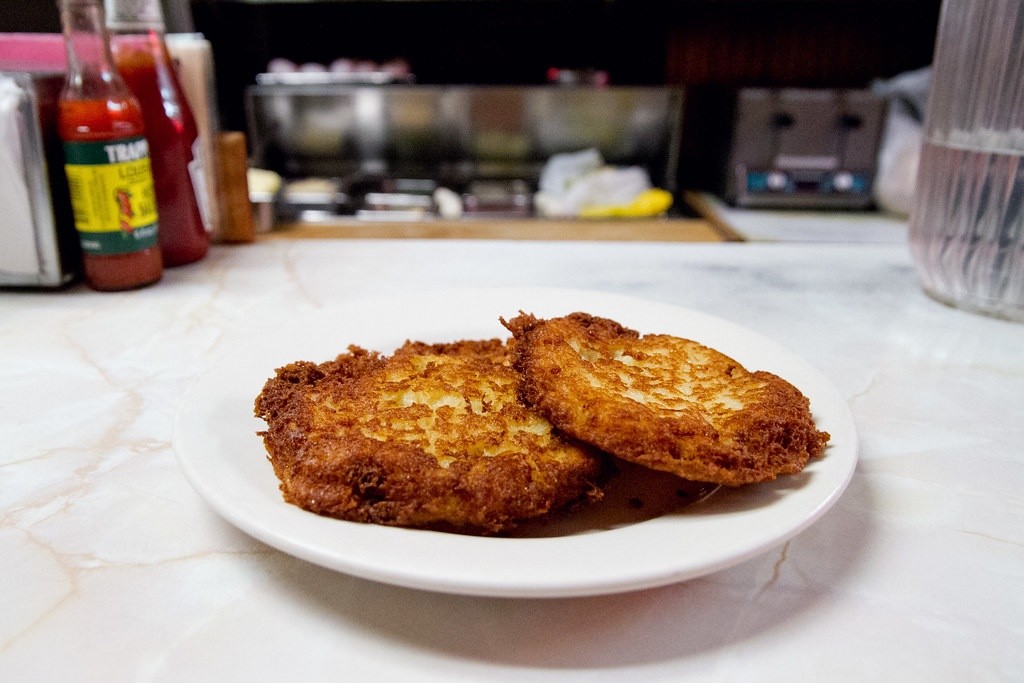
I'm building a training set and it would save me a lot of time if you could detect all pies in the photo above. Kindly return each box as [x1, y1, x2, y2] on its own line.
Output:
[247, 337, 615, 535]
[495, 309, 831, 485]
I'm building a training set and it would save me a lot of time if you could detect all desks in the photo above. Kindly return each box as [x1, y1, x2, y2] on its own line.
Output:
[0, 237, 1024, 683]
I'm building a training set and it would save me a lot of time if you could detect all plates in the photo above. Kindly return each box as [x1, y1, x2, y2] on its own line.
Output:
[171, 289, 862, 599]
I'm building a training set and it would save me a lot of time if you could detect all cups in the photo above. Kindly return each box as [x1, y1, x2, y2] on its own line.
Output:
[902, 0, 1023, 320]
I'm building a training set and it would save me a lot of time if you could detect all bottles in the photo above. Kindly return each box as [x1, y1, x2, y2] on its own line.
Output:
[54, 0, 164, 292]
[102, 0, 212, 267]
